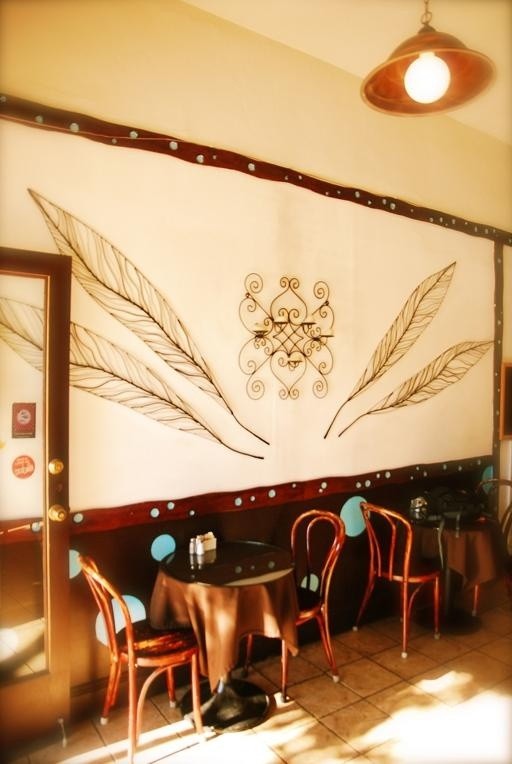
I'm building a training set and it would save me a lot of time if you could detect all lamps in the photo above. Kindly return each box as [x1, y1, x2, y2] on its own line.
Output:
[360, 0, 498, 118]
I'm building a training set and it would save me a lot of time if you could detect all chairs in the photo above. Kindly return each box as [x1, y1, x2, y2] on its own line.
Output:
[465, 479, 512, 617]
[76, 555, 205, 764]
[352, 502, 442, 659]
[244, 510, 345, 702]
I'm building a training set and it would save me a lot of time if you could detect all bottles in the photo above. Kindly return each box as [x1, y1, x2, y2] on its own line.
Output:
[197, 555, 205, 570]
[189, 554, 195, 570]
[189, 538, 195, 554]
[195, 540, 206, 555]
